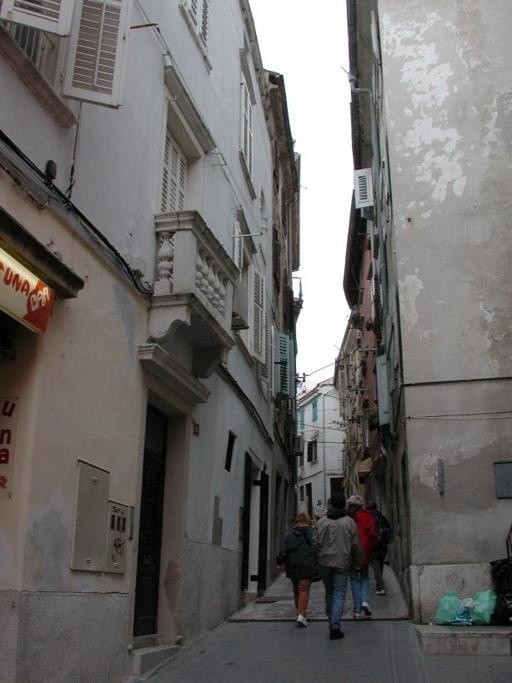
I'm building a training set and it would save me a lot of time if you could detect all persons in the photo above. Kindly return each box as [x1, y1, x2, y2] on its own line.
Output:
[308, 493, 362, 639]
[365, 500, 393, 595]
[345, 494, 381, 620]
[275, 510, 322, 626]
[312, 498, 328, 521]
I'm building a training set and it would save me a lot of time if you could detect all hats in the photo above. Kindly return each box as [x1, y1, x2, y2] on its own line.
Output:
[346, 495, 363, 505]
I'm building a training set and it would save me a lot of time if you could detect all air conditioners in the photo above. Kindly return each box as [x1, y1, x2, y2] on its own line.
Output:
[297, 436, 304, 456]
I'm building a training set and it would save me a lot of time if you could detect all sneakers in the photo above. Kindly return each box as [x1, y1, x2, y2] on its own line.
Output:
[376, 590, 384, 595]
[297, 614, 309, 626]
[354, 601, 373, 617]
[330, 630, 344, 639]
